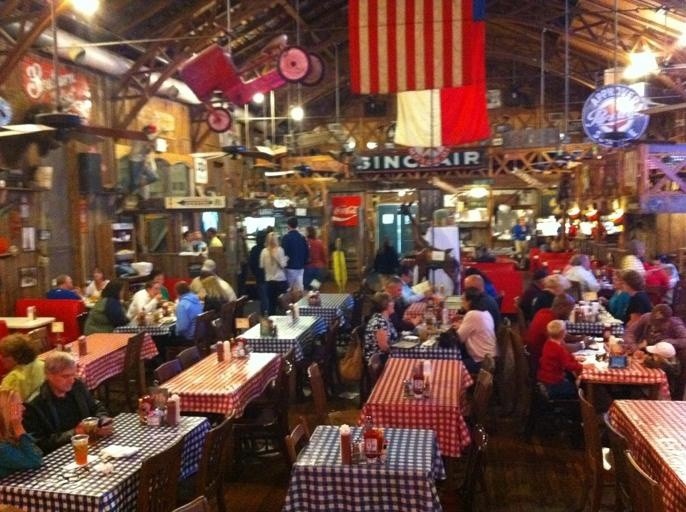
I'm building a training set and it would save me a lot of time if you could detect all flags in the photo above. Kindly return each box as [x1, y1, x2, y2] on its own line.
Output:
[390, 0, 489, 148]
[347, 0, 476, 98]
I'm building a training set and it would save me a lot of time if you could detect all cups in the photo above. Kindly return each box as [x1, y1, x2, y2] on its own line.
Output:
[72, 432, 89, 466]
[83, 415, 99, 444]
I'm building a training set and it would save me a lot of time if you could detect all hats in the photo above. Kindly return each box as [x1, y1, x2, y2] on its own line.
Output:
[201, 259, 218, 273]
[646, 341, 676, 358]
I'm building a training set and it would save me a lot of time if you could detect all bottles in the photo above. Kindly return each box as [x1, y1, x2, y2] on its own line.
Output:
[27, 304, 36, 320]
[413, 360, 424, 398]
[78, 335, 87, 355]
[339, 422, 352, 464]
[260, 309, 273, 337]
[574, 307, 586, 323]
[286, 302, 299, 323]
[424, 376, 432, 398]
[308, 290, 321, 305]
[603, 323, 612, 342]
[216, 340, 230, 362]
[56, 338, 65, 352]
[361, 414, 384, 458]
[402, 379, 410, 397]
[139, 392, 181, 427]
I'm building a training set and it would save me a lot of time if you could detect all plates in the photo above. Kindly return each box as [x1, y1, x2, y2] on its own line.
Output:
[403, 335, 418, 340]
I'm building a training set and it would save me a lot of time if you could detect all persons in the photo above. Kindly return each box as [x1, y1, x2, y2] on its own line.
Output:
[509, 218, 610, 267]
[355, 238, 502, 396]
[0, 384, 42, 480]
[232, 216, 328, 320]
[521, 237, 686, 409]
[25, 351, 107, 451]
[47, 227, 238, 369]
[0, 331, 46, 405]
